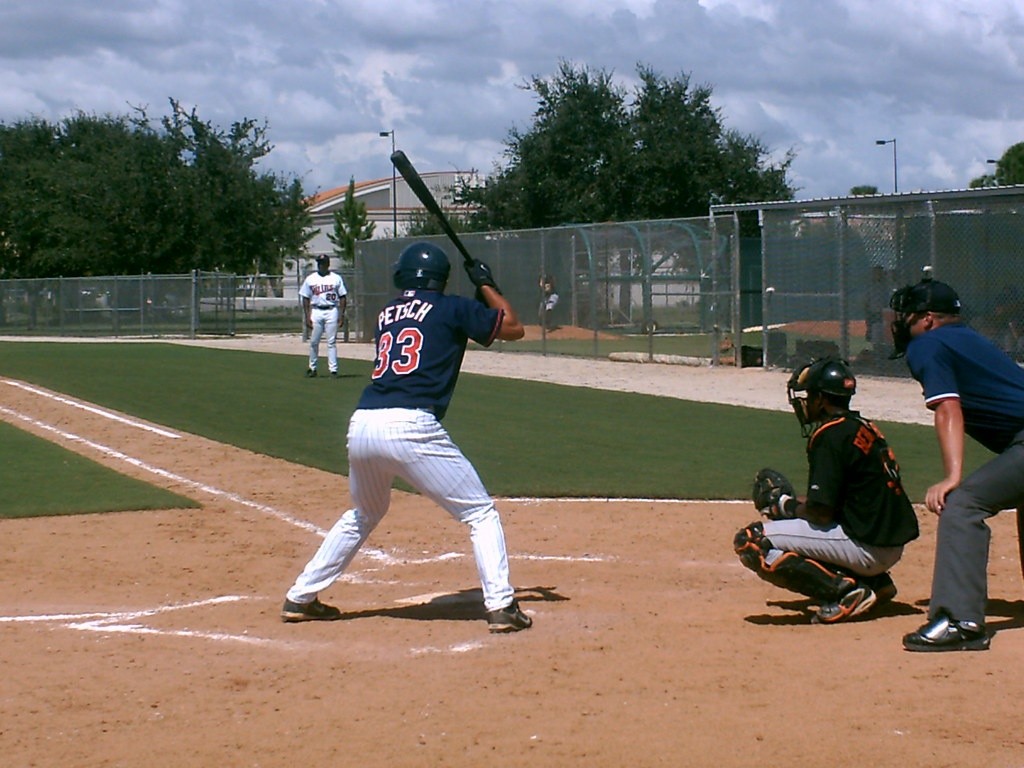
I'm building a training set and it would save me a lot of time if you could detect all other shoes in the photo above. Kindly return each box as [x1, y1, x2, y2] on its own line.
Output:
[304, 369, 317, 378]
[328, 371, 338, 381]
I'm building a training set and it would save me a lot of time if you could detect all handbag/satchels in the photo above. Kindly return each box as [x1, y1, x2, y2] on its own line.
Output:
[785, 339, 841, 369]
[735, 345, 762, 368]
[710, 335, 736, 366]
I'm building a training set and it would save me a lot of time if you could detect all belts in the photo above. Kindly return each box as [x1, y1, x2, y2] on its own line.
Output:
[317, 305, 335, 310]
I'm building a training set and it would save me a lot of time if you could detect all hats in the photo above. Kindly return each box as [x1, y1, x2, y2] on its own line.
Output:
[315, 254, 330, 263]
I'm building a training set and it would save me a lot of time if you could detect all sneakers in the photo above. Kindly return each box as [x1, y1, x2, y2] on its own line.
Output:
[856, 572, 897, 610]
[281, 597, 340, 622]
[903, 613, 990, 652]
[487, 598, 532, 632]
[819, 581, 878, 625]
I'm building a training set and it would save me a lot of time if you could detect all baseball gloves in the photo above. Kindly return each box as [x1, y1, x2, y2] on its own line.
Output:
[751, 467, 797, 519]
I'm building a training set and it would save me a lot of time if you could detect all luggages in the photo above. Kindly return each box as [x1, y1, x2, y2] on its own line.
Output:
[763, 327, 787, 369]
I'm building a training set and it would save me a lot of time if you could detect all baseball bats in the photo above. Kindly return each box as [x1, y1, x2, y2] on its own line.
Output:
[390, 149, 473, 265]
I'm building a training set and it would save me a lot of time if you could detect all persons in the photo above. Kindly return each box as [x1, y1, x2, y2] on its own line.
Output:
[863, 265, 884, 350]
[994, 284, 1024, 369]
[889, 279, 1024, 651]
[298, 254, 347, 379]
[280, 242, 532, 634]
[538, 273, 560, 332]
[733, 358, 920, 625]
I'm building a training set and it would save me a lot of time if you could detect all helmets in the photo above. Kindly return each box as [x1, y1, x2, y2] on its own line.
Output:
[392, 242, 451, 293]
[889, 277, 960, 314]
[787, 355, 856, 397]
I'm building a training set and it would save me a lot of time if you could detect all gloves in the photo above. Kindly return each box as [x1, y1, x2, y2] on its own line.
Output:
[463, 257, 503, 308]
